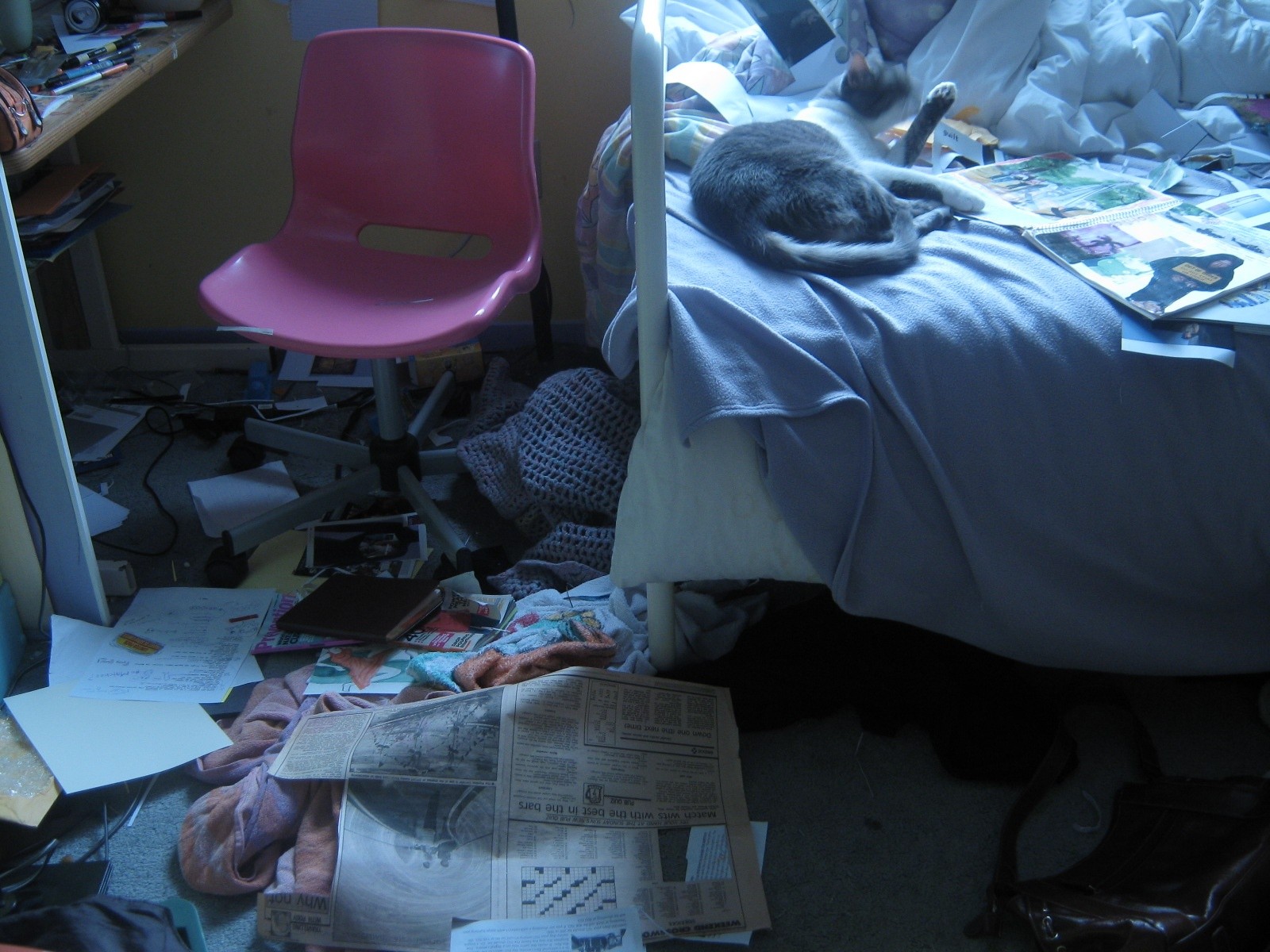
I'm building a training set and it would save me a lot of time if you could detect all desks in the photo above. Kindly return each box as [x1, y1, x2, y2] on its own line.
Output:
[0, 0, 271, 397]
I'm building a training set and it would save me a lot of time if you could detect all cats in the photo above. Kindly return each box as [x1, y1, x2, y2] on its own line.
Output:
[687, 51, 986, 277]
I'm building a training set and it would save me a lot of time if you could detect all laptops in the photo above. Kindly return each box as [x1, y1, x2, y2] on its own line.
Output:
[0, 860, 114, 899]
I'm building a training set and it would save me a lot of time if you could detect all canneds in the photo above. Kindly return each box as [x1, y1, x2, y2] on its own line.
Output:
[64, 0, 114, 34]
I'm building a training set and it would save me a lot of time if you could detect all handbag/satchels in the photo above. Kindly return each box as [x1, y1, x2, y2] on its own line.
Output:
[0, 67, 43, 155]
[981, 685, 1270, 952]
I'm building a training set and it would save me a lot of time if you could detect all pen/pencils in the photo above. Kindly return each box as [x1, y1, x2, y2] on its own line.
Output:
[117, 10, 203, 21]
[29, 33, 141, 97]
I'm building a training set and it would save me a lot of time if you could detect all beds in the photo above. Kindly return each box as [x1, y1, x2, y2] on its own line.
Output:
[600, 1, 1270, 680]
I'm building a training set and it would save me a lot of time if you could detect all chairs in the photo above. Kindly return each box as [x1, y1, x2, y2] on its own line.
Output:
[197, 28, 543, 586]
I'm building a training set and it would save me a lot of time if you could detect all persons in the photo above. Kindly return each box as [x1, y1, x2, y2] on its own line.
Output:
[1126, 253, 1244, 313]
[1181, 322, 1200, 339]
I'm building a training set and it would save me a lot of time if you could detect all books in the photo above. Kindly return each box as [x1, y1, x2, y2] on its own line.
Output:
[249, 589, 518, 655]
[937, 150, 1270, 338]
